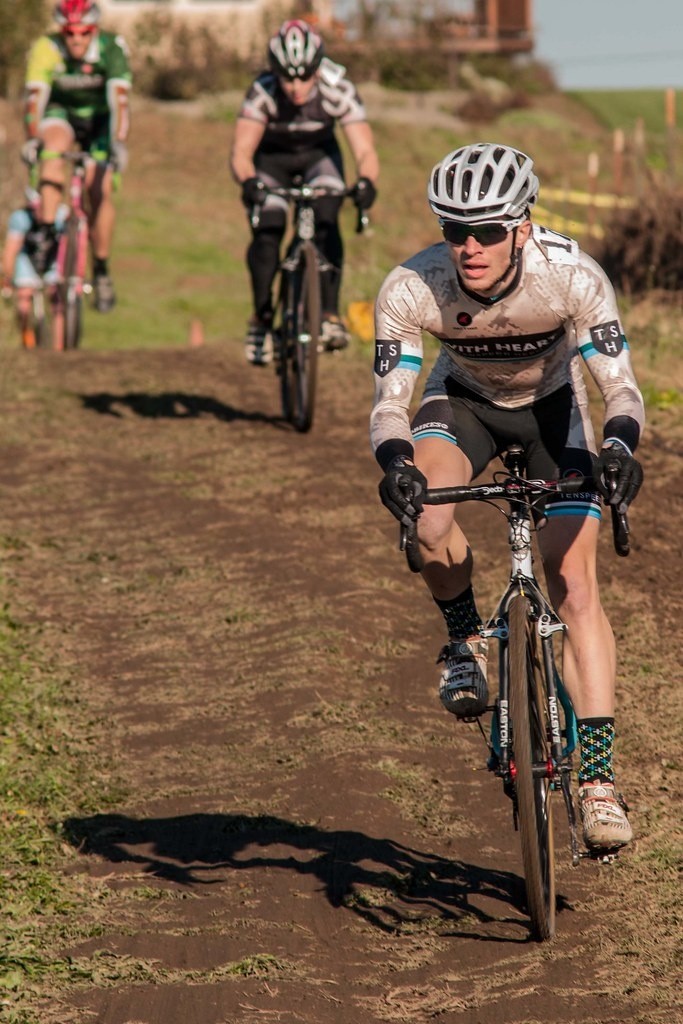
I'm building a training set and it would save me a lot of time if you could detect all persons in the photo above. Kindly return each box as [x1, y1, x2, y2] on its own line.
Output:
[230, 19, 378, 367]
[0, 190, 71, 334]
[368, 143, 644, 845]
[21, 0, 132, 311]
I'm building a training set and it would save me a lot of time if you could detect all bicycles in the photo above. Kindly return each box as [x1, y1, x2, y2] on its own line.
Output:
[391, 445, 635, 943]
[242, 180, 370, 434]
[3, 144, 121, 353]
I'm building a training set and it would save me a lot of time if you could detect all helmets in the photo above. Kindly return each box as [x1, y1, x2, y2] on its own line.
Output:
[267, 19, 324, 81]
[53, 0, 100, 25]
[428, 143, 539, 222]
[25, 185, 41, 208]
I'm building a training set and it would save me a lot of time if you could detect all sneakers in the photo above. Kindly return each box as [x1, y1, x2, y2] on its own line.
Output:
[245, 316, 274, 366]
[318, 318, 351, 349]
[435, 635, 488, 716]
[572, 779, 633, 849]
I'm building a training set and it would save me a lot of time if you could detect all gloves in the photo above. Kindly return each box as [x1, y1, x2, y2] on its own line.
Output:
[22, 137, 40, 166]
[594, 434, 644, 514]
[352, 176, 376, 209]
[109, 139, 127, 177]
[242, 178, 270, 209]
[374, 456, 427, 527]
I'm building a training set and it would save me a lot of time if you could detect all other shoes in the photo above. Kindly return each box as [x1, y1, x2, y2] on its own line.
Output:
[93, 274, 113, 312]
[30, 224, 55, 271]
[23, 323, 37, 348]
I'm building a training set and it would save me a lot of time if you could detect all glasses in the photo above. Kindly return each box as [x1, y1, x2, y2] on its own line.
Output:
[438, 211, 528, 246]
[60, 25, 94, 36]
